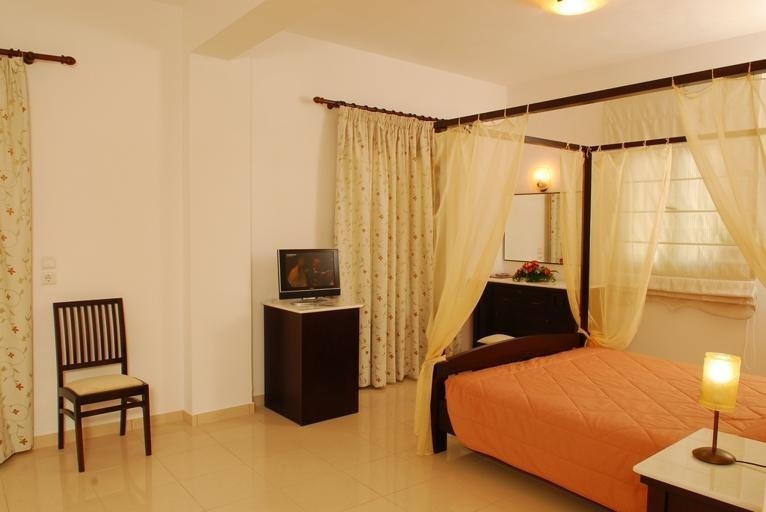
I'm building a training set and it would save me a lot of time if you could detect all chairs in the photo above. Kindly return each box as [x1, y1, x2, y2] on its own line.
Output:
[52, 297, 152, 471]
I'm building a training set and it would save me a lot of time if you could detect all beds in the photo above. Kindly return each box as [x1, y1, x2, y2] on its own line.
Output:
[430, 333, 766, 511]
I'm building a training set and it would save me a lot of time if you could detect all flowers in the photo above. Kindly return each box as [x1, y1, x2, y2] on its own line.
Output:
[511, 260, 559, 283]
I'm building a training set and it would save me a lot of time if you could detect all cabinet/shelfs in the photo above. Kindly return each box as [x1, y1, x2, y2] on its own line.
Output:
[262, 301, 365, 426]
[474, 275, 606, 349]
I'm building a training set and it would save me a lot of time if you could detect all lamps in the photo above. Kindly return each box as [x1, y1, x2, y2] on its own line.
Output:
[691, 351, 742, 465]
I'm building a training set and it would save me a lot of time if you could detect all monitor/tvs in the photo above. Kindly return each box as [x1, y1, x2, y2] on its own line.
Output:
[277, 249, 340, 305]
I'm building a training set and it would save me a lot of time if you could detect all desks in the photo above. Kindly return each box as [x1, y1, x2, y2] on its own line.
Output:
[632, 427, 766, 512]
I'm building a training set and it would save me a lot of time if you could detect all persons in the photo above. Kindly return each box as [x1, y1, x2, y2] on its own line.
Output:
[308, 258, 325, 287]
[286, 254, 307, 287]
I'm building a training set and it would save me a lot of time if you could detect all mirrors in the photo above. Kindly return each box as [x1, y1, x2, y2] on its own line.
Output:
[503, 192, 582, 264]
